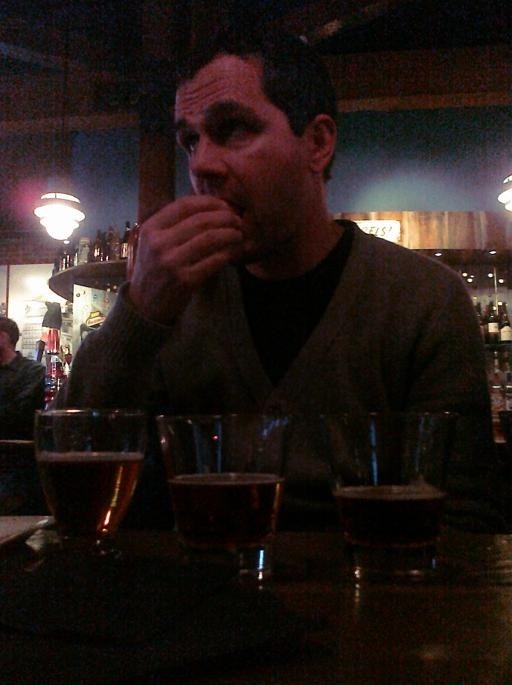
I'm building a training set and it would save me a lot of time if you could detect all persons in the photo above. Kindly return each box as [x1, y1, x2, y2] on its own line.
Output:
[46, 20, 505, 529]
[1, 316, 46, 439]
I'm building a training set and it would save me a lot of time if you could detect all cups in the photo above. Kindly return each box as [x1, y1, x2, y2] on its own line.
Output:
[29, 402, 143, 589]
[156, 407, 296, 591]
[317, 400, 456, 586]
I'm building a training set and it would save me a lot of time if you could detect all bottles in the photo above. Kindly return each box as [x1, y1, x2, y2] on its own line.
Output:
[476, 300, 511, 435]
[52, 220, 132, 273]
